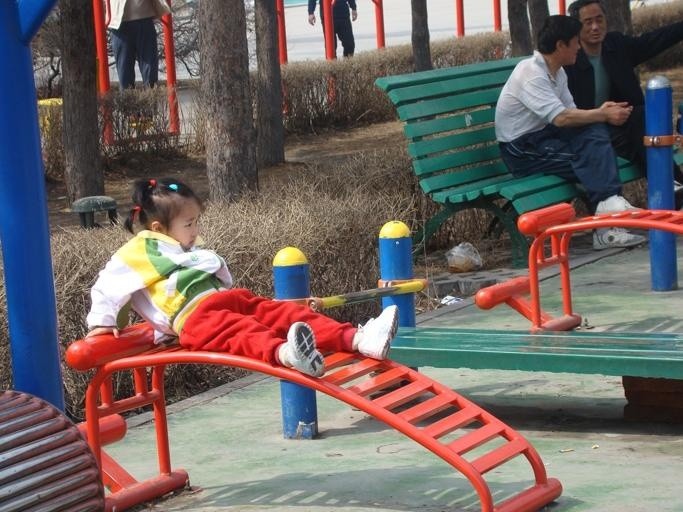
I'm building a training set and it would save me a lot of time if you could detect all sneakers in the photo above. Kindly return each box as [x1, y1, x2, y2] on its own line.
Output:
[592, 225, 646, 251]
[279, 321, 326, 378]
[594, 194, 644, 216]
[352, 304, 399, 361]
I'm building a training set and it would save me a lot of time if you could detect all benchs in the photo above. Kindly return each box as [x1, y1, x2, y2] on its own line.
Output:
[372, 55, 682, 268]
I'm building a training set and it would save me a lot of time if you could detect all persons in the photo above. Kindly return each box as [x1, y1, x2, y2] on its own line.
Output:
[495, 15, 645, 248]
[559, 1, 682, 211]
[106, 0, 171, 91]
[85, 177, 399, 377]
[308, 0, 357, 59]
